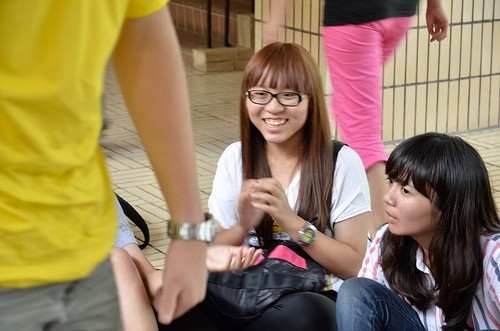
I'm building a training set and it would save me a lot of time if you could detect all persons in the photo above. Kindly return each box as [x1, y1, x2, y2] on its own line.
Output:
[157, 42, 373, 330]
[335, 132, 500, 331]
[109, 194, 165, 331]
[0, 0, 217, 331]
[322, 0, 448, 243]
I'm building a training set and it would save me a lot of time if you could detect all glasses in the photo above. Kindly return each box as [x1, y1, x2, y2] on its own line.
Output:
[244, 89, 305, 107]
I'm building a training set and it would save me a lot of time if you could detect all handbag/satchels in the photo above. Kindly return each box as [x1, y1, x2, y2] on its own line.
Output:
[206, 139, 348, 325]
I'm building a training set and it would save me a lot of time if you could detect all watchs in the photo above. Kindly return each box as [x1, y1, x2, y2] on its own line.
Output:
[166, 212, 216, 244]
[291, 220, 317, 247]
